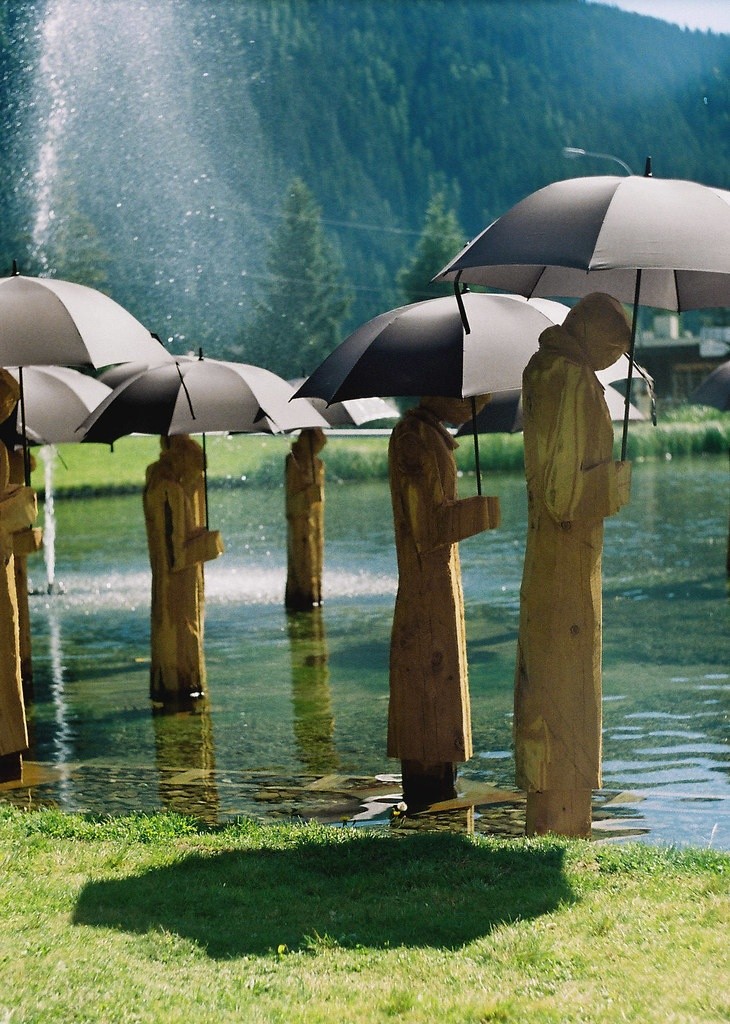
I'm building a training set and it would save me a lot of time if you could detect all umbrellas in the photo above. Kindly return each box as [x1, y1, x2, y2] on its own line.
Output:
[690, 361, 730, 412]
[73, 348, 335, 533]
[101, 355, 218, 388]
[226, 370, 403, 487]
[431, 154, 730, 462]
[288, 286, 607, 497]
[5, 367, 115, 444]
[0, 258, 147, 489]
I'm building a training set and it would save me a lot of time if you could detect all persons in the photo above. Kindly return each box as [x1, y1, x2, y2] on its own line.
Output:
[520, 291, 632, 838]
[0, 369, 47, 784]
[384, 389, 500, 821]
[142, 434, 221, 702]
[286, 428, 327, 607]
